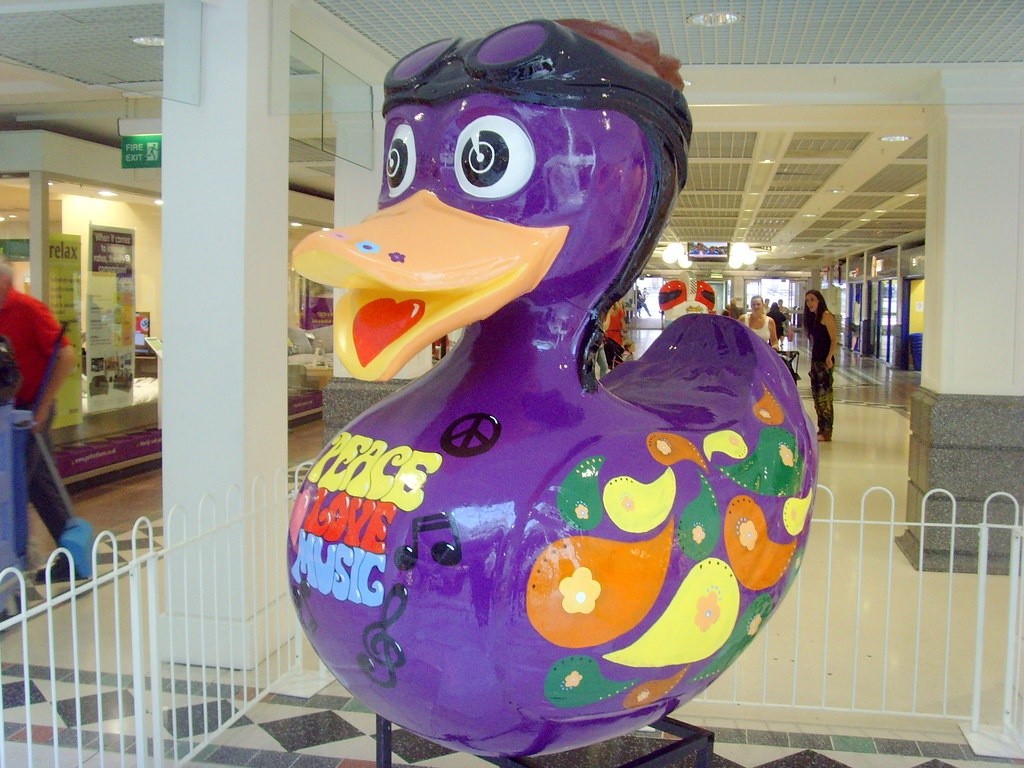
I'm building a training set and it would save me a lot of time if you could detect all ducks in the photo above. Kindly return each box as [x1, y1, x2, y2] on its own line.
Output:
[284, 19, 817, 756]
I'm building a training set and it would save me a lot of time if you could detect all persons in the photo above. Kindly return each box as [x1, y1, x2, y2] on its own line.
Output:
[1, 261, 77, 582]
[802, 289, 837, 442]
[596, 282, 653, 378]
[689, 242, 728, 256]
[723, 293, 797, 352]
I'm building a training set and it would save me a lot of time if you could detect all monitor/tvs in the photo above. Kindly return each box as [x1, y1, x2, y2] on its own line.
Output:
[688, 241, 730, 262]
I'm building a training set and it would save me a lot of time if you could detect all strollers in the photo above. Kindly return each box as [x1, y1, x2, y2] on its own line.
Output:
[602, 335, 625, 369]
[776, 351, 801, 388]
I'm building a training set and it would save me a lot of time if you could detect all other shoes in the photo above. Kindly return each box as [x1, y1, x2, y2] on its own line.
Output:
[818, 434, 831, 441]
[36, 559, 88, 583]
